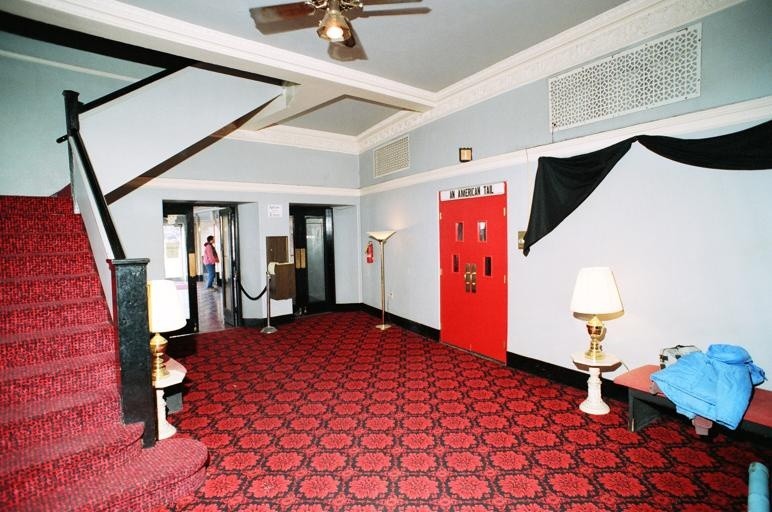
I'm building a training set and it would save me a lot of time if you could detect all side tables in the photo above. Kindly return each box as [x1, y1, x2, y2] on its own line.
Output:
[574, 354, 621, 416]
[151, 357, 187, 441]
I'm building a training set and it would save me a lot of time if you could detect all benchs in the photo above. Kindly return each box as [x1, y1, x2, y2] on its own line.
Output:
[611, 364, 772, 440]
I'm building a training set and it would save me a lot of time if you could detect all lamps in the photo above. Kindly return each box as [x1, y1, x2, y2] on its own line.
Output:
[316, 0, 351, 43]
[367, 231, 395, 331]
[146, 280, 187, 381]
[571, 266, 624, 358]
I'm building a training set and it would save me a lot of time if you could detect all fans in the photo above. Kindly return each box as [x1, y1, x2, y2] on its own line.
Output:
[249, 0, 431, 62]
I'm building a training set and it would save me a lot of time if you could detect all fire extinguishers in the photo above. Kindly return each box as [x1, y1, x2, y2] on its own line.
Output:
[365, 241, 373, 263]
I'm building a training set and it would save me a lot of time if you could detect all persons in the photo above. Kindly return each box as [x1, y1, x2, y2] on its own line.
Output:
[203, 235, 220, 292]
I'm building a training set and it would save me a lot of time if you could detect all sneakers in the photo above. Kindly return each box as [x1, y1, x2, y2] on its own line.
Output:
[207, 288, 218, 292]
